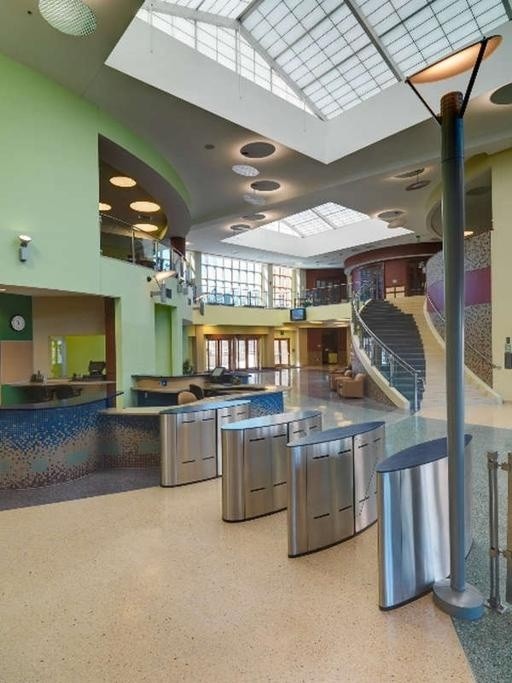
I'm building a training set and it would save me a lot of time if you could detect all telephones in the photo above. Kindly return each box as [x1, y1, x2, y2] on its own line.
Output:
[32, 370, 44, 382]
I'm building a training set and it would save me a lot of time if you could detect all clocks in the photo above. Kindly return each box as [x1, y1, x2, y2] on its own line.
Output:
[10, 313, 26, 332]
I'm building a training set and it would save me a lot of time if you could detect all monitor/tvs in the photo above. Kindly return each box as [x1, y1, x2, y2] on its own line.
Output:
[290, 308, 306, 321]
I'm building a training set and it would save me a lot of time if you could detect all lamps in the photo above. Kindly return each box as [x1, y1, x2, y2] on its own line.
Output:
[193, 294, 207, 316]
[151, 270, 176, 304]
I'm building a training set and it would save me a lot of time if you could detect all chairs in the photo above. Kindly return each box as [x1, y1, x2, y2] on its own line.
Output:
[55, 383, 73, 400]
[328, 365, 366, 400]
[177, 391, 197, 406]
[189, 383, 204, 400]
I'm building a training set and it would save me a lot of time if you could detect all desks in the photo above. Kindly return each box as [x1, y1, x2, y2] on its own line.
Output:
[9, 374, 114, 401]
